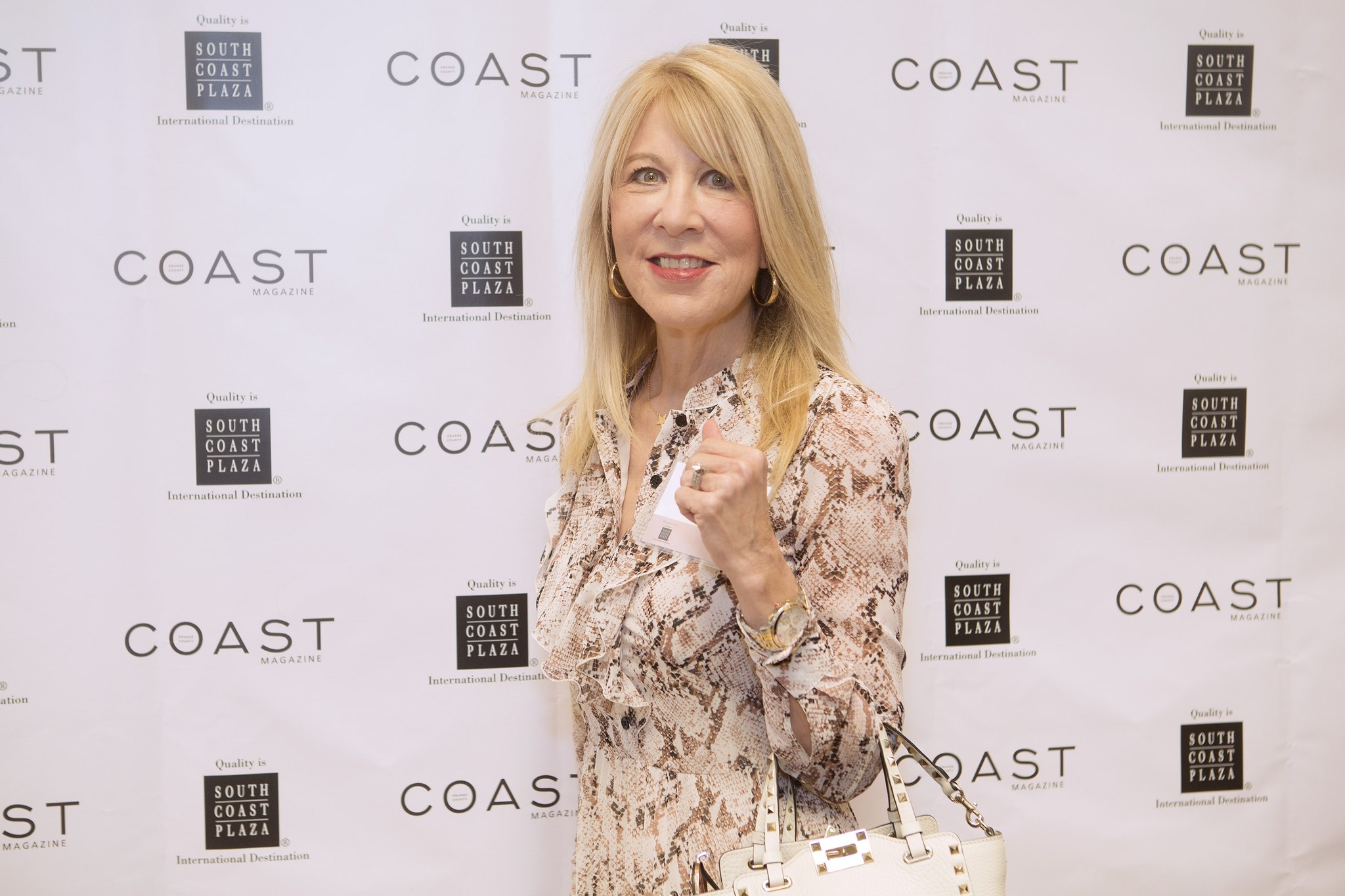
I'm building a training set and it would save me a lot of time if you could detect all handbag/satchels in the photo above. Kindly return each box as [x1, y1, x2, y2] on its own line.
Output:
[691, 714, 1008, 896]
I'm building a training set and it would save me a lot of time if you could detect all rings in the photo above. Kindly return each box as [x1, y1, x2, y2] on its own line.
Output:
[692, 463, 715, 490]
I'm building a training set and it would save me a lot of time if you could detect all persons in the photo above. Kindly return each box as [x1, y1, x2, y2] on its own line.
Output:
[532, 43, 910, 896]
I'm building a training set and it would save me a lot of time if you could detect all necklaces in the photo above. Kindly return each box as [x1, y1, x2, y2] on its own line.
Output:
[648, 351, 668, 429]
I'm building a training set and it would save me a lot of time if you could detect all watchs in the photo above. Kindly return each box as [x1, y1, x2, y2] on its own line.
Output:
[740, 582, 809, 652]
